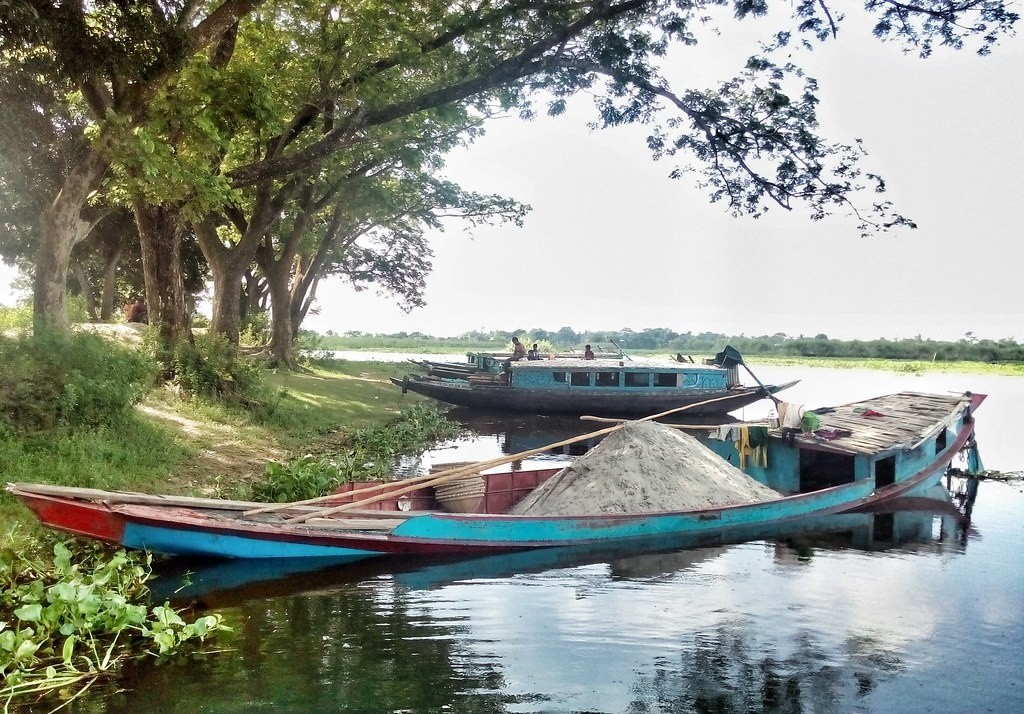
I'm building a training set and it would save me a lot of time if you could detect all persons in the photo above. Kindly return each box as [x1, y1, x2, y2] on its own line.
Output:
[527, 344, 540, 360]
[500, 337, 525, 375]
[129, 297, 147, 322]
[585, 345, 594, 360]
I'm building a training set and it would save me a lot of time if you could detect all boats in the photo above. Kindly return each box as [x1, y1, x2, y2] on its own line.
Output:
[389, 351, 803, 414]
[5, 389, 990, 565]
